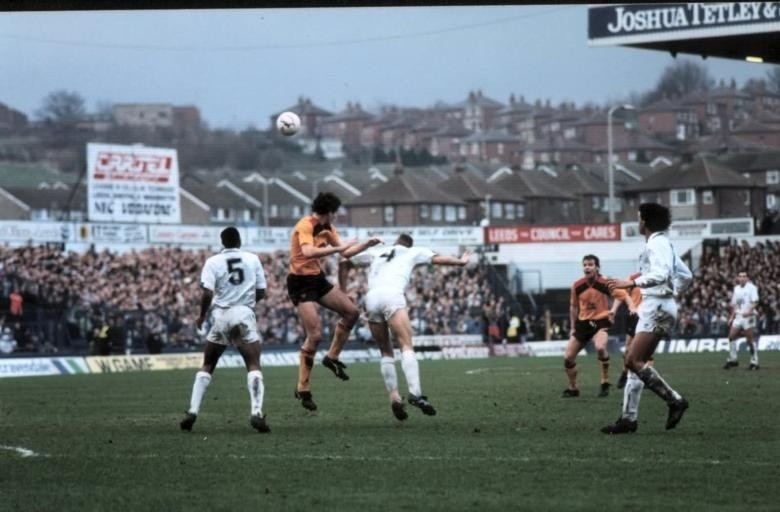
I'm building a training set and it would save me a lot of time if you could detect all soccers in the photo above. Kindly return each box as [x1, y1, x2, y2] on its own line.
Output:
[276, 111, 300, 135]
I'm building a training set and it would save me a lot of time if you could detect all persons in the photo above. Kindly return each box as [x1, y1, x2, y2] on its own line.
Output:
[564, 256, 642, 399]
[668, 238, 780, 370]
[287, 192, 385, 410]
[255, 251, 371, 343]
[339, 233, 474, 420]
[1, 237, 210, 359]
[600, 203, 689, 434]
[407, 250, 571, 357]
[180, 227, 270, 432]
[607, 273, 655, 388]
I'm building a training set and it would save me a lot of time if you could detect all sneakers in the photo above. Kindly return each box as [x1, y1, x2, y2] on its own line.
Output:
[408, 393, 436, 415]
[722, 360, 740, 370]
[178, 412, 196, 432]
[248, 413, 271, 435]
[598, 381, 610, 397]
[748, 363, 759, 370]
[601, 418, 639, 435]
[293, 386, 318, 411]
[667, 397, 688, 432]
[323, 356, 350, 381]
[561, 388, 580, 397]
[392, 399, 408, 420]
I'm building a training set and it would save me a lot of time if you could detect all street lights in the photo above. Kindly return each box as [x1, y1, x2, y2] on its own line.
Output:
[607, 100, 639, 222]
